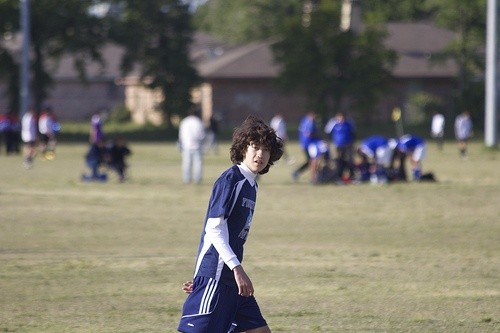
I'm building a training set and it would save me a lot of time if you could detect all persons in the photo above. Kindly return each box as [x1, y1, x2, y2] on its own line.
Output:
[175, 114, 284, 332]
[19, 106, 38, 168]
[431, 109, 446, 151]
[178, 106, 205, 186]
[291, 110, 427, 186]
[38, 105, 58, 157]
[455, 109, 473, 157]
[271, 112, 297, 166]
